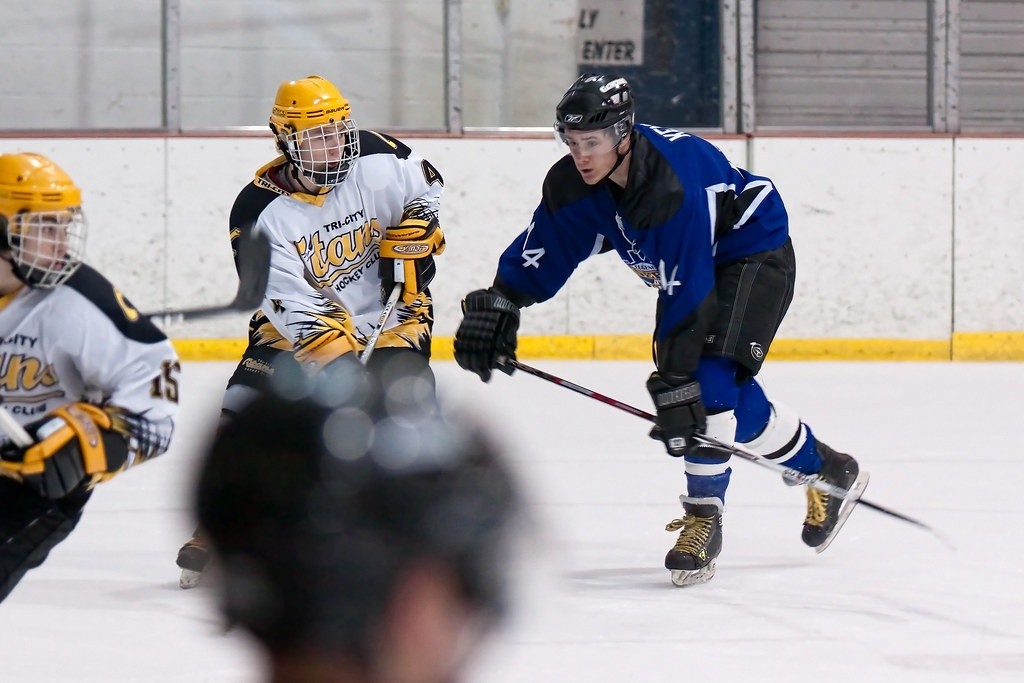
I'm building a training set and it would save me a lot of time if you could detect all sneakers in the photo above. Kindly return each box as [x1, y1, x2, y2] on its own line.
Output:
[176, 523, 214, 589]
[664, 494, 724, 586]
[801, 434, 870, 554]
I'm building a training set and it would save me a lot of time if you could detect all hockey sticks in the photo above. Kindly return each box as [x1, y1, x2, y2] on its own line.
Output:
[498, 355, 932, 536]
[0, 406, 34, 448]
[359, 280, 406, 363]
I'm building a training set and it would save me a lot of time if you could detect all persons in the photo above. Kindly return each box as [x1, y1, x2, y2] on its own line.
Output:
[177, 76, 446, 590]
[193, 369, 519, 683]
[0, 150, 180, 600]
[454, 71, 869, 588]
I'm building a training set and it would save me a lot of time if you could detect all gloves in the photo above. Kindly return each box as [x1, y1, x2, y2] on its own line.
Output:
[378, 224, 436, 308]
[647, 371, 707, 457]
[453, 289, 520, 383]
[0, 402, 128, 499]
[300, 334, 365, 380]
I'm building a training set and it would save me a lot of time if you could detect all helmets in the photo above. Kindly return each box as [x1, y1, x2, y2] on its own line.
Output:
[197, 380, 512, 612]
[270, 76, 351, 183]
[0, 152, 82, 289]
[555, 72, 634, 131]
